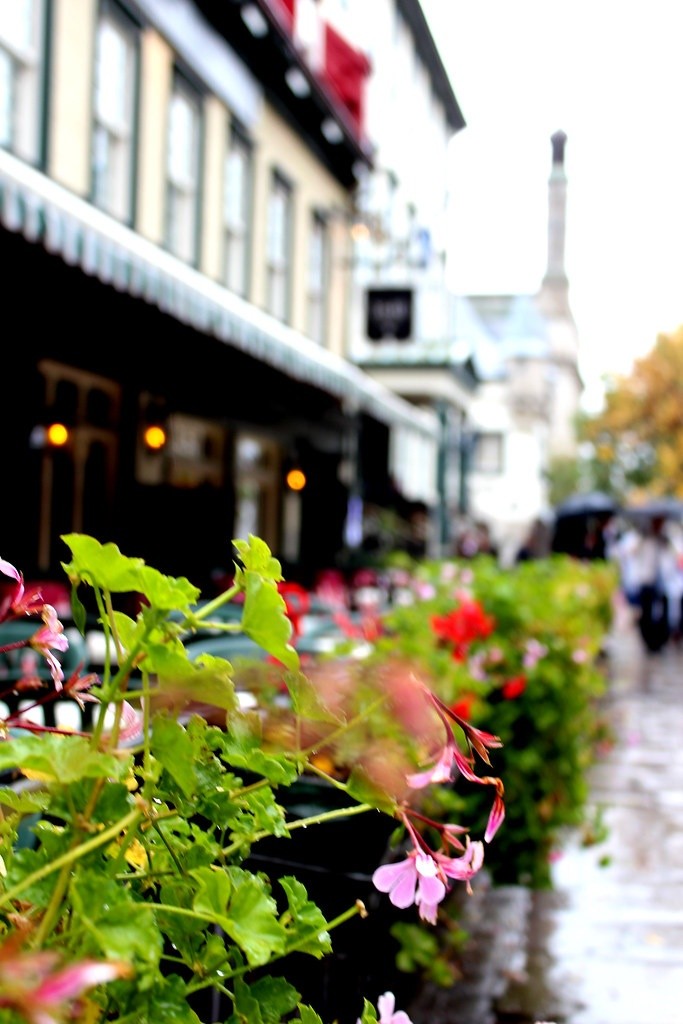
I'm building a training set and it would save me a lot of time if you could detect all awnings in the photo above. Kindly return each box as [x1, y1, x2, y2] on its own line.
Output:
[0, 147, 442, 445]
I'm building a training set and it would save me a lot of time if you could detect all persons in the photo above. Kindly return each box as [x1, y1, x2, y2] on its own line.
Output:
[428, 501, 682, 644]
